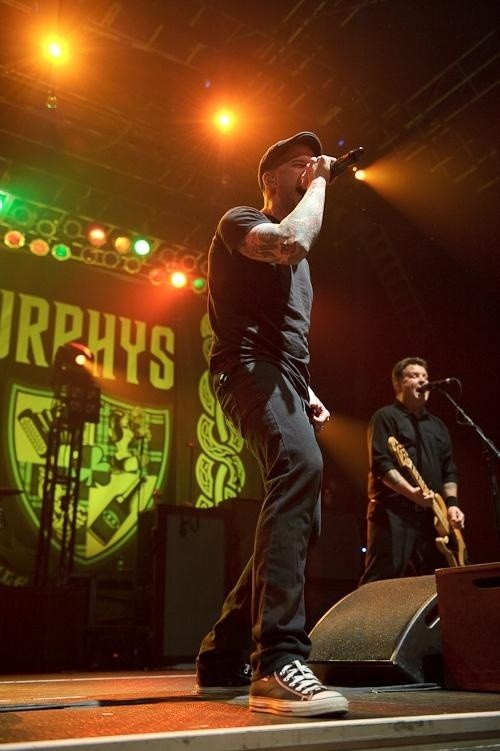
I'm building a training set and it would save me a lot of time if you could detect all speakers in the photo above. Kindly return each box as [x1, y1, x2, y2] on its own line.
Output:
[435, 562, 500, 693]
[303, 574, 440, 687]
[136, 504, 234, 665]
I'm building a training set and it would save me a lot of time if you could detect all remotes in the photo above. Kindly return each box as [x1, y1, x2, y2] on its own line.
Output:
[423, 377, 454, 391]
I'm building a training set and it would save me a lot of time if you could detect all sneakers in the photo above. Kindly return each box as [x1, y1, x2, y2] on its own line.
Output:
[249, 659, 349, 717]
[196, 662, 251, 694]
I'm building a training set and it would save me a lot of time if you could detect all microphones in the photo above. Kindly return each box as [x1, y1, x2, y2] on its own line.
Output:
[329, 147, 363, 183]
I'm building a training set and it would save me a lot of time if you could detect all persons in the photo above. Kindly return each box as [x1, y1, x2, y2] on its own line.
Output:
[194, 128, 352, 719]
[356, 357, 469, 587]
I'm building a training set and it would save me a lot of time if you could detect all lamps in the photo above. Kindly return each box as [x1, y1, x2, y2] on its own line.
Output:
[0, 157, 210, 302]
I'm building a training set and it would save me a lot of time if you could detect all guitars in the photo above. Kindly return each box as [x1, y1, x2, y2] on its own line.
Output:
[387, 436, 466, 567]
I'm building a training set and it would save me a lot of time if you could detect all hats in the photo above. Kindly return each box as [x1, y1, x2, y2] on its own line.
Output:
[257, 131, 322, 190]
